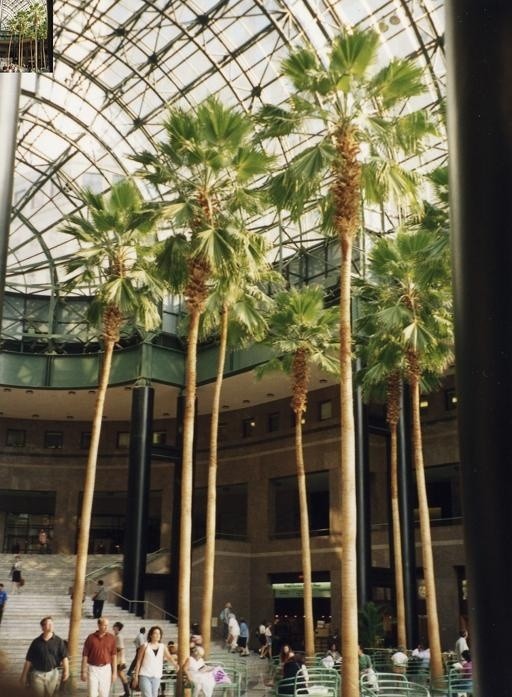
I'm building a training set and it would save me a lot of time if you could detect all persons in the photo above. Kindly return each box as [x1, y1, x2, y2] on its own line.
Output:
[112, 621, 131, 696]
[167, 637, 215, 697]
[127, 625, 147, 675]
[257, 619, 272, 660]
[20, 615, 70, 696]
[8, 554, 25, 594]
[455, 630, 472, 681]
[91, 580, 108, 615]
[1, 582, 8, 623]
[67, 578, 88, 606]
[266, 644, 309, 694]
[358, 644, 379, 692]
[80, 616, 118, 696]
[11, 525, 127, 553]
[391, 642, 430, 685]
[325, 643, 342, 667]
[131, 625, 179, 696]
[224, 602, 250, 657]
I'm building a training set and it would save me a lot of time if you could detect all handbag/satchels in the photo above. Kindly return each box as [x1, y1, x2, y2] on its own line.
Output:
[130, 675, 140, 690]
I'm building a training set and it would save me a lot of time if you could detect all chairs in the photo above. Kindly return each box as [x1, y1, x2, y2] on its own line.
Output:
[149, 641, 477, 696]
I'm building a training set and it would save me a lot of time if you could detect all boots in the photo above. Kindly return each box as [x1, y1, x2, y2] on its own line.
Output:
[119, 681, 130, 696]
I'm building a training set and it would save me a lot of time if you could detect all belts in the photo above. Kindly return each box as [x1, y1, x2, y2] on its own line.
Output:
[92, 662, 105, 666]
